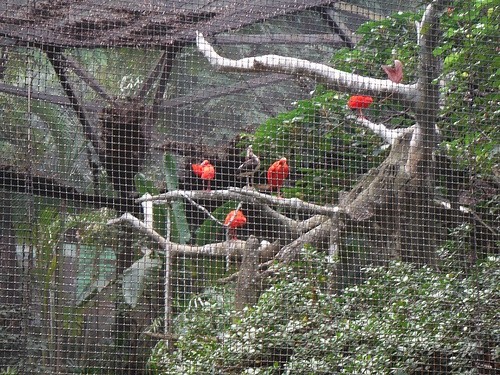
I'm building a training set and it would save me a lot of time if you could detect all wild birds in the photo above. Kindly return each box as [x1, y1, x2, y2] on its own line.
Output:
[192, 159, 216, 191]
[267, 157, 289, 196]
[381, 60, 404, 84]
[224, 210, 247, 240]
[348, 95, 374, 119]
[236, 144, 261, 189]
